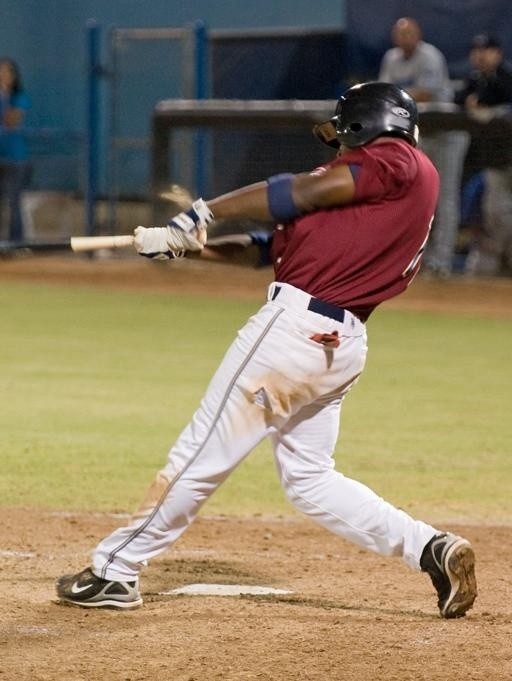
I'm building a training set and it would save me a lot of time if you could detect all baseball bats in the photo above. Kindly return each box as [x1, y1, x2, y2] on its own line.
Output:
[0, 235, 136, 263]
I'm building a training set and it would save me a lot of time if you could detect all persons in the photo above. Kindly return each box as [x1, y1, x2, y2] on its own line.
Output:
[455, 32, 511, 279]
[51, 80, 478, 623]
[376, 14, 472, 279]
[1, 55, 37, 264]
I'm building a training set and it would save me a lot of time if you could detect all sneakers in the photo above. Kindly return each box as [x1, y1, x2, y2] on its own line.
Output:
[419, 531, 480, 620]
[55, 566, 144, 609]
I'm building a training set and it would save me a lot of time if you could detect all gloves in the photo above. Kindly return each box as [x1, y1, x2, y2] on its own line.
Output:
[166, 197, 215, 252]
[133, 225, 188, 262]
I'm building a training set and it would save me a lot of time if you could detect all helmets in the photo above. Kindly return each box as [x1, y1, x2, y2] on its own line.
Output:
[312, 81, 421, 149]
